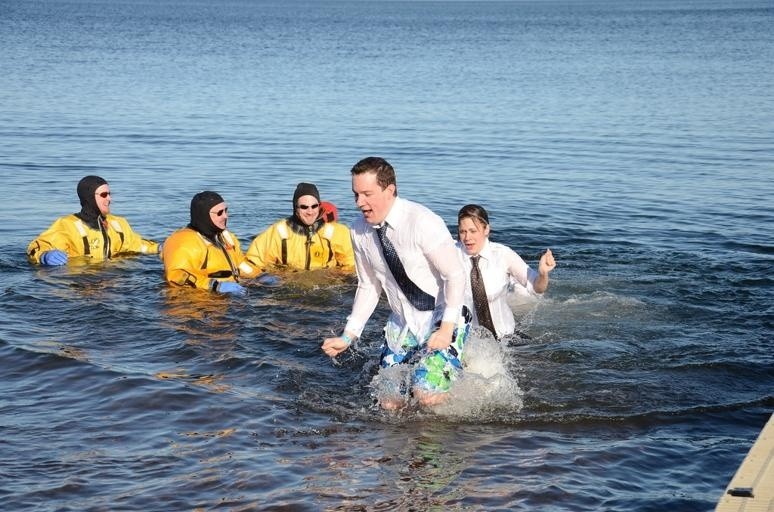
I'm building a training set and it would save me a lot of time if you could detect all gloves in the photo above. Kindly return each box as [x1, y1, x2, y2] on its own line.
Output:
[210, 280, 247, 297]
[40, 251, 68, 266]
[256, 272, 281, 285]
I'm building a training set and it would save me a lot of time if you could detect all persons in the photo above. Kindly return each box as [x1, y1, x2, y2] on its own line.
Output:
[160, 191, 280, 297]
[455, 204, 556, 341]
[247, 183, 354, 275]
[527, 254, 531, 254]
[321, 157, 473, 413]
[26, 176, 163, 266]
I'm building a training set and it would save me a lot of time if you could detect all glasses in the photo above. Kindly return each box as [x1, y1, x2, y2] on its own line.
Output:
[298, 204, 318, 209]
[210, 207, 227, 216]
[95, 192, 110, 198]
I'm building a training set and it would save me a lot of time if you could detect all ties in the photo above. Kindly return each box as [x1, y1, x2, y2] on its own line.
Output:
[376, 224, 436, 312]
[470, 255, 497, 340]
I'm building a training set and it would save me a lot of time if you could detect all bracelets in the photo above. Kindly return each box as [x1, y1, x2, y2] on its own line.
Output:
[340, 334, 352, 345]
